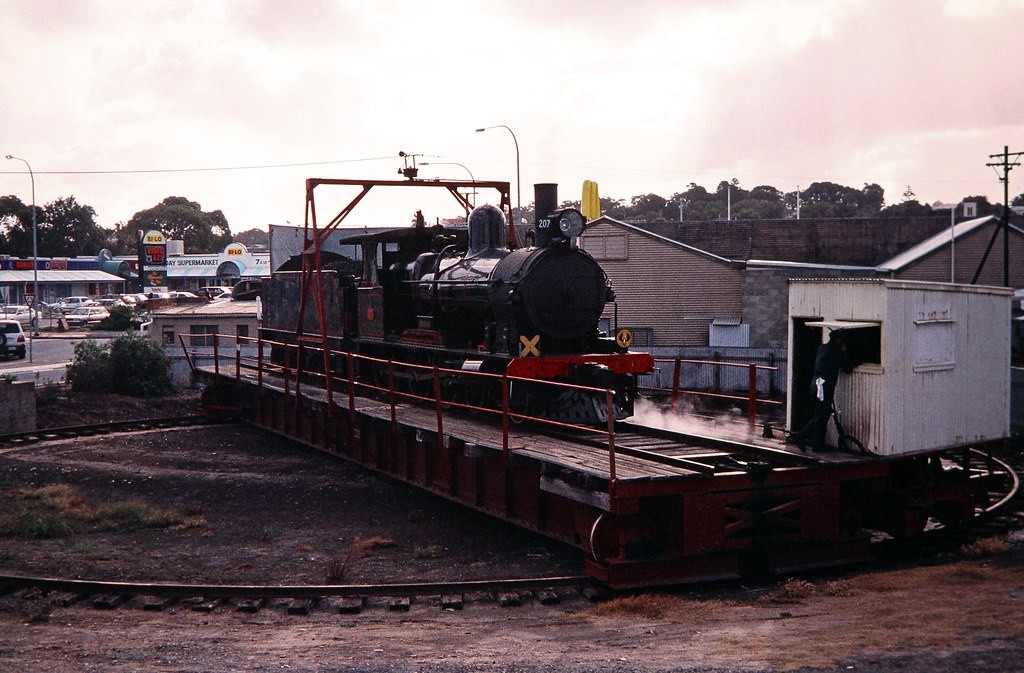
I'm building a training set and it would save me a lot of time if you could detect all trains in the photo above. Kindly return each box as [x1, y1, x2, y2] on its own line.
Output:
[266, 183, 656, 433]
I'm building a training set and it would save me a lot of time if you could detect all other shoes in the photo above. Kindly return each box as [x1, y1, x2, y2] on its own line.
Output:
[812, 445, 826, 452]
[792, 432, 806, 452]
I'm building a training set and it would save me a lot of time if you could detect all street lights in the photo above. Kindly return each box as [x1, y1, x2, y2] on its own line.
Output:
[419, 161, 476, 211]
[6, 155, 39, 337]
[477, 125, 523, 224]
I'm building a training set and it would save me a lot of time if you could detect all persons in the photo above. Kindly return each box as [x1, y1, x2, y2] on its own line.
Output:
[792, 330, 856, 452]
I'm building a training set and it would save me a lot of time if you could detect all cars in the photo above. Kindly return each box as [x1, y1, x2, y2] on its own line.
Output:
[0, 286, 234, 360]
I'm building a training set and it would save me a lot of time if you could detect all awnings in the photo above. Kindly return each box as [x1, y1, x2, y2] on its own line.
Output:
[0, 270, 127, 305]
